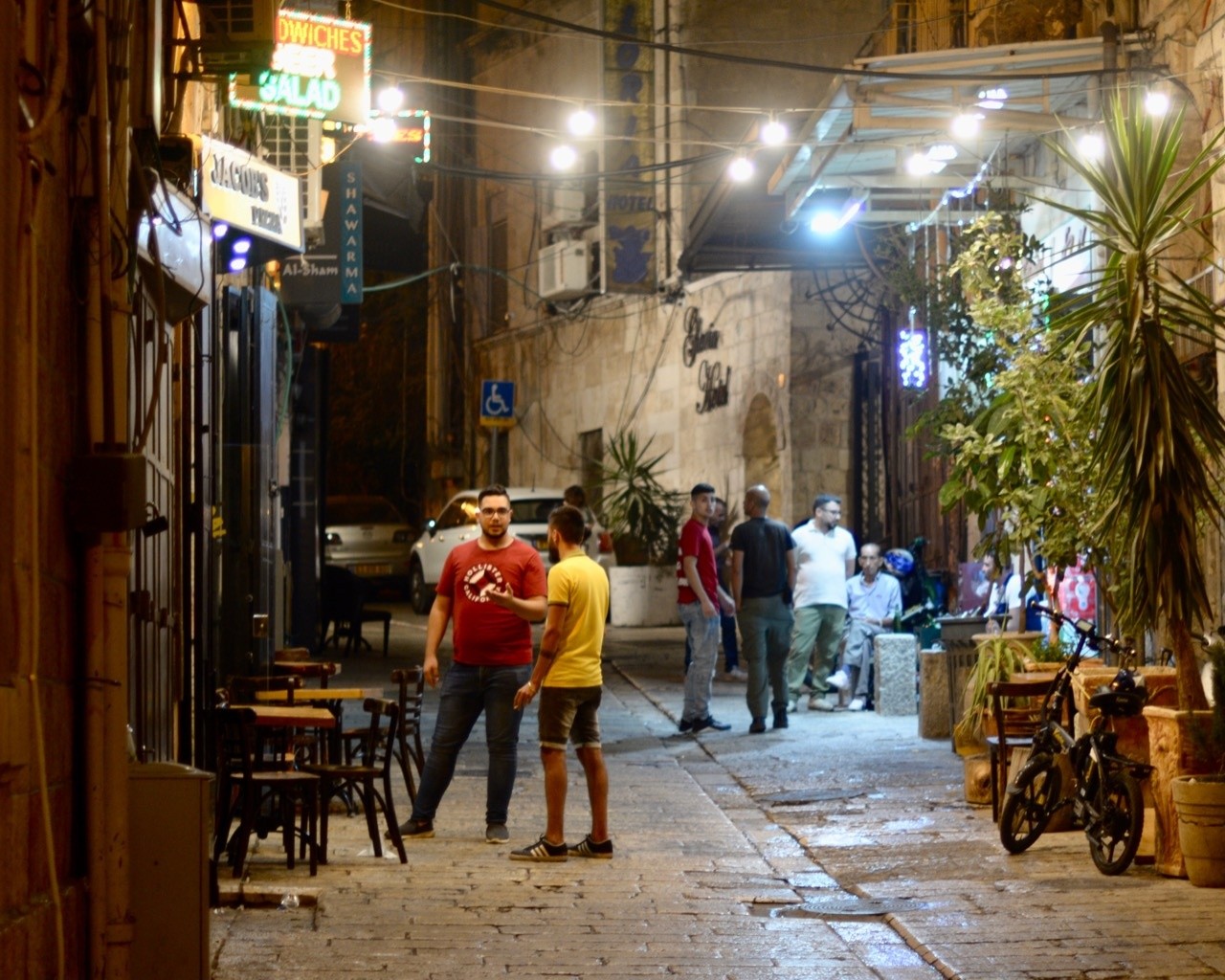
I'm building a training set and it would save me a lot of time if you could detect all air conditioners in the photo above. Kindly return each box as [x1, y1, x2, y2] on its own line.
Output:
[257, 111, 323, 228]
[539, 168, 585, 234]
[538, 238, 590, 301]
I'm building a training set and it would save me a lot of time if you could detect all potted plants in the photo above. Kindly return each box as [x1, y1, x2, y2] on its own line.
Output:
[591, 432, 689, 628]
[936, 69, 1225, 879]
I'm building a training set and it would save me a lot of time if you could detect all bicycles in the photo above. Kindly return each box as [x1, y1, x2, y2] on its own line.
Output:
[997, 598, 1156, 876]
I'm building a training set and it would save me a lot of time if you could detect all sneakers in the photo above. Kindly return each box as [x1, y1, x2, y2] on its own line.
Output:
[568, 835, 616, 860]
[507, 833, 569, 862]
[384, 818, 435, 841]
[484, 823, 511, 846]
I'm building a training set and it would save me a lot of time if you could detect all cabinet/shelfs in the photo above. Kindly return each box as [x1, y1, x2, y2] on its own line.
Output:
[129, 759, 216, 980]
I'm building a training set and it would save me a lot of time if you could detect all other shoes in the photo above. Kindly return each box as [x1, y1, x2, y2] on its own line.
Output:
[786, 699, 799, 713]
[679, 715, 696, 733]
[747, 720, 768, 735]
[773, 716, 789, 729]
[806, 697, 834, 713]
[695, 715, 734, 736]
[825, 669, 853, 692]
[724, 668, 748, 682]
[847, 697, 866, 711]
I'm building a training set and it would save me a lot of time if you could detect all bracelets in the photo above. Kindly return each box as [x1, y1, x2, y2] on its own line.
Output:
[528, 681, 538, 694]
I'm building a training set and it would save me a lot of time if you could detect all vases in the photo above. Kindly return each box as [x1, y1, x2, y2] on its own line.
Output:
[1170, 773, 1225, 888]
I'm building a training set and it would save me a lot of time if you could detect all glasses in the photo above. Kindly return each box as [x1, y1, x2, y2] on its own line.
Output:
[482, 506, 510, 519]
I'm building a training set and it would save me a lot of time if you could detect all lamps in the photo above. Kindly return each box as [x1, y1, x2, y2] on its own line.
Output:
[907, 82, 1008, 173]
[808, 190, 872, 242]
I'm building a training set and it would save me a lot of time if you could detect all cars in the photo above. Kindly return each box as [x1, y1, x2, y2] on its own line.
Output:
[407, 485, 617, 623]
[322, 492, 417, 594]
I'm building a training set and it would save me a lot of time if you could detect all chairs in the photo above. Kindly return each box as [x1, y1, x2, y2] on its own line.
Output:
[316, 564, 393, 661]
[212, 648, 428, 881]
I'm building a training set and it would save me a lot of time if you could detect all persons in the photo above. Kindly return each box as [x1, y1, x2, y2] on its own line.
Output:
[983, 551, 1042, 637]
[675, 483, 901, 734]
[383, 484, 547, 843]
[560, 486, 605, 564]
[509, 505, 613, 860]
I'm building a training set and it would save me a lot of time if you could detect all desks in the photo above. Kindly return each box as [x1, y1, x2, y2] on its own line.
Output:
[275, 661, 342, 763]
[229, 707, 336, 862]
[254, 685, 383, 781]
